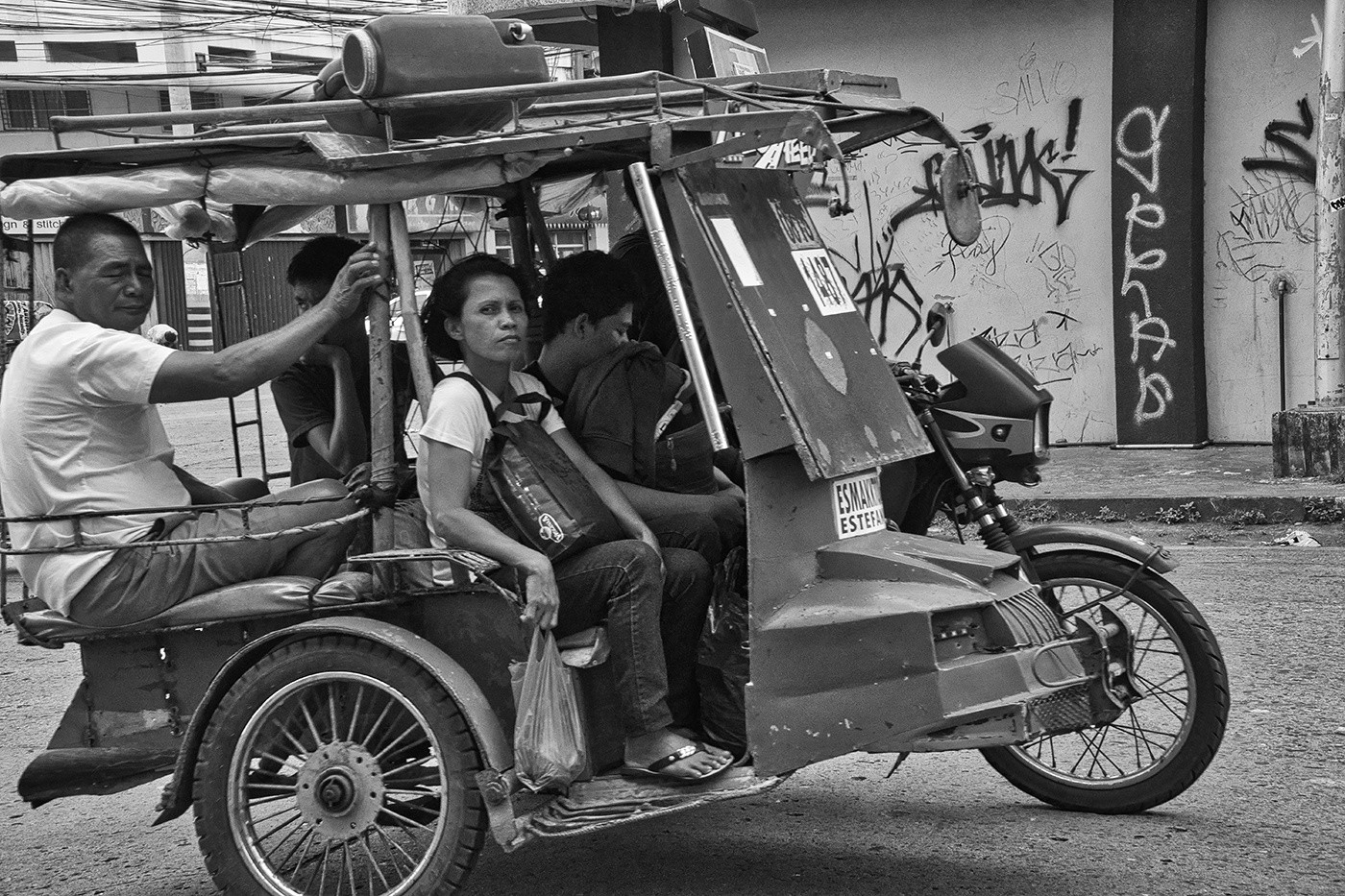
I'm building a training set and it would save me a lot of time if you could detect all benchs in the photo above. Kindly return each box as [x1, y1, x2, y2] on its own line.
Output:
[392, 497, 702, 776]
[18, 561, 377, 645]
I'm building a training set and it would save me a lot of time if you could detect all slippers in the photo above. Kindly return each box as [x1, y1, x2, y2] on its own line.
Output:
[619, 736, 734, 780]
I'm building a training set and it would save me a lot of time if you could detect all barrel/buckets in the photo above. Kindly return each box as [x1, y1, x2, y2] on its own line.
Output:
[312, 13, 548, 136]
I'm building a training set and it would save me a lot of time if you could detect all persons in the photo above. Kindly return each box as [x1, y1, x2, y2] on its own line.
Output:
[0, 210, 359, 630]
[270, 231, 443, 489]
[612, 164, 707, 343]
[422, 252, 736, 781]
[524, 250, 746, 564]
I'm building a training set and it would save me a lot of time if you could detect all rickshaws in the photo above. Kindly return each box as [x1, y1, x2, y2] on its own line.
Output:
[2, 6, 1233, 896]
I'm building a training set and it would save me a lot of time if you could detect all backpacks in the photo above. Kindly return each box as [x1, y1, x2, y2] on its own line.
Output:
[563, 338, 716, 495]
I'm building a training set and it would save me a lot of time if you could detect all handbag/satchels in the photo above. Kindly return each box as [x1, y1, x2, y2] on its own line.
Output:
[694, 546, 750, 749]
[469, 392, 602, 561]
[508, 624, 586, 794]
